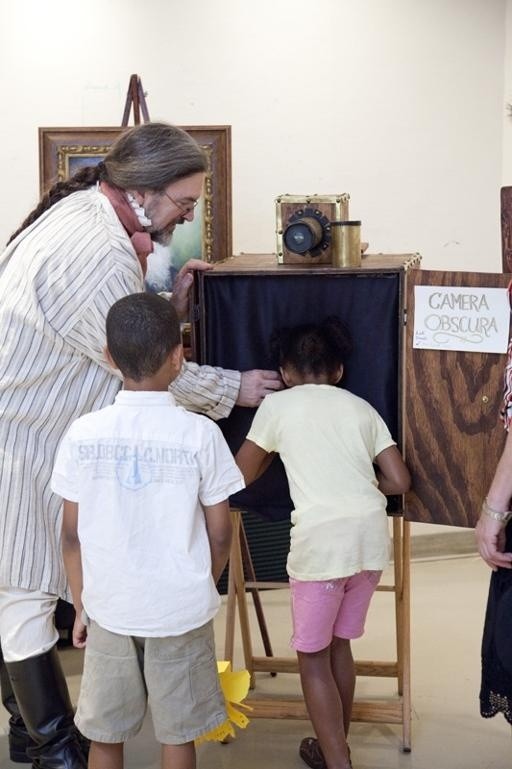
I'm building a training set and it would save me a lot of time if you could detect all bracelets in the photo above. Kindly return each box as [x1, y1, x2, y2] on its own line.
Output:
[480, 497, 508, 527]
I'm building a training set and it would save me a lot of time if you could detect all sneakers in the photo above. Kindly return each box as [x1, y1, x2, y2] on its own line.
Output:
[299, 738, 352, 769]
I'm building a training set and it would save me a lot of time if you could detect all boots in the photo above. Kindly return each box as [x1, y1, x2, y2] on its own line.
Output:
[0, 643, 90, 769]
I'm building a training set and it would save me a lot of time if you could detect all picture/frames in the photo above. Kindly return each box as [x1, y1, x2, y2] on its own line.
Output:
[39, 124, 234, 362]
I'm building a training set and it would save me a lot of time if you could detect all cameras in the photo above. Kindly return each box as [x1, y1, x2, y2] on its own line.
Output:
[276, 194, 349, 265]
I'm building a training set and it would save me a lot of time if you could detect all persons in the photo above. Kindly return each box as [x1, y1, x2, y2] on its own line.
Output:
[238, 318, 412, 767]
[1, 121, 283, 767]
[472, 269, 512, 728]
[49, 291, 249, 766]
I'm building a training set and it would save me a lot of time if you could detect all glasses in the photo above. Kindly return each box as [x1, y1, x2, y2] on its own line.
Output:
[163, 190, 198, 218]
